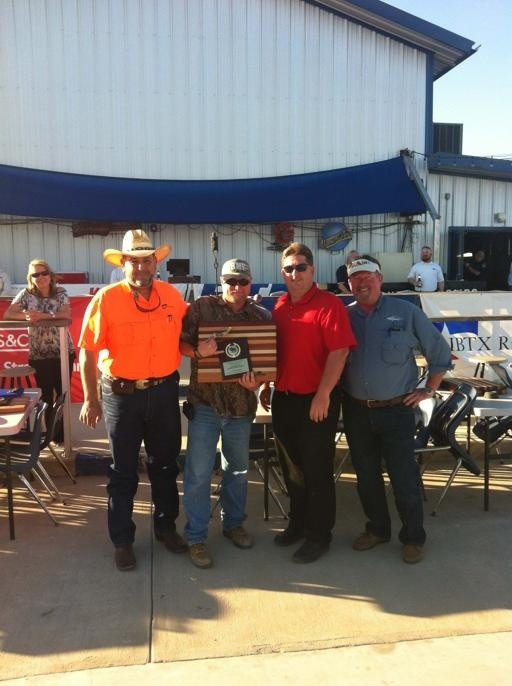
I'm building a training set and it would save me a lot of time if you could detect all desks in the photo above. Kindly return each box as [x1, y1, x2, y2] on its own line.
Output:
[414, 350, 507, 378]
[252, 402, 290, 523]
[0, 386, 42, 541]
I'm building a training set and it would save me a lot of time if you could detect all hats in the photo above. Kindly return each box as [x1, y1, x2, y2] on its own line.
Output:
[103, 228, 172, 267]
[347, 259, 382, 277]
[221, 259, 251, 277]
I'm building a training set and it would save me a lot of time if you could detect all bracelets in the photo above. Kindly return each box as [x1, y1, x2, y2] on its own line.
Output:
[194, 351, 202, 359]
[49, 311, 56, 320]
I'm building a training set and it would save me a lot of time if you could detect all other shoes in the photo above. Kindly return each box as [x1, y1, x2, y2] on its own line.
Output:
[115, 543, 136, 571]
[158, 531, 187, 553]
[292, 538, 330, 564]
[274, 526, 304, 547]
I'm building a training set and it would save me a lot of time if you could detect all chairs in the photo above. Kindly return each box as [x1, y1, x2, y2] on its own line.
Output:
[1, 402, 64, 525]
[412, 382, 480, 518]
[4, 392, 76, 506]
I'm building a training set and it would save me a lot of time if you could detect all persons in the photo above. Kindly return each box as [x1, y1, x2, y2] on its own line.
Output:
[336, 250, 358, 294]
[179, 258, 277, 568]
[466, 250, 487, 280]
[110, 267, 126, 284]
[76, 228, 189, 572]
[259, 242, 358, 566]
[3, 258, 76, 445]
[407, 247, 445, 291]
[338, 255, 452, 563]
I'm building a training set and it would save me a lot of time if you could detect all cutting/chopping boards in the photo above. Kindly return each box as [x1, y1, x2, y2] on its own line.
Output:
[0, 395, 31, 413]
[443, 376, 509, 394]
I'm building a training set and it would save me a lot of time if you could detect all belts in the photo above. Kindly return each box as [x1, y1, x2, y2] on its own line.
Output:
[340, 390, 412, 408]
[102, 369, 180, 391]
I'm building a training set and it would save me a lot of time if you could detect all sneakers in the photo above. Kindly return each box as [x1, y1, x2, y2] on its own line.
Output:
[222, 527, 253, 550]
[190, 543, 212, 568]
[402, 544, 423, 564]
[353, 532, 390, 551]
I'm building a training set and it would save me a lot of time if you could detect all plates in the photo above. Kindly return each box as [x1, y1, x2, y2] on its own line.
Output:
[1, 387, 26, 397]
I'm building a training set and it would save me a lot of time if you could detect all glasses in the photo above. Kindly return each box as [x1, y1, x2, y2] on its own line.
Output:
[283, 264, 309, 273]
[223, 278, 251, 286]
[32, 271, 49, 278]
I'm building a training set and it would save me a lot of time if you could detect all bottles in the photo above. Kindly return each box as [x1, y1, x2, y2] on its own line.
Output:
[156, 271, 160, 281]
[417, 273, 422, 287]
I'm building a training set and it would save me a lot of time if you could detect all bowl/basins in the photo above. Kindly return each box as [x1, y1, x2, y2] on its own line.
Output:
[483, 392, 499, 399]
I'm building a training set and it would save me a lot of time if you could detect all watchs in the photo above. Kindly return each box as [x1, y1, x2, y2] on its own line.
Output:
[423, 387, 433, 396]
